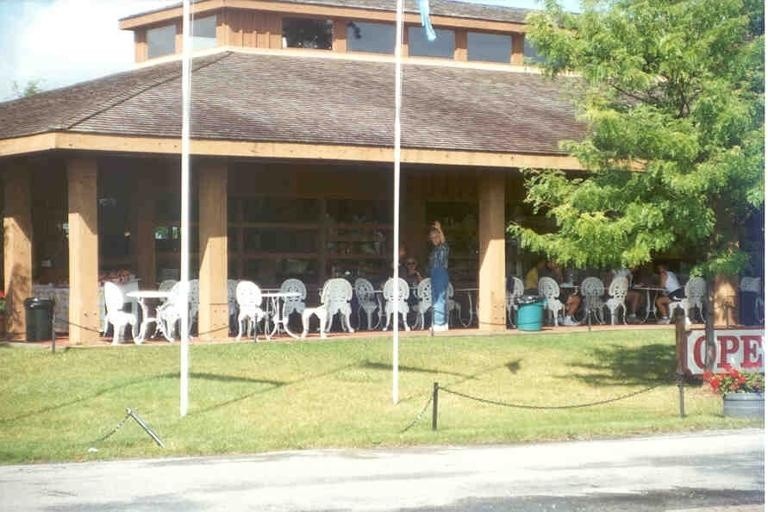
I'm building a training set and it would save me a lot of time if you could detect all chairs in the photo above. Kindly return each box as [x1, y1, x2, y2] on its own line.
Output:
[226, 276, 459, 340]
[159, 280, 176, 302]
[188, 279, 199, 334]
[666, 276, 707, 325]
[506, 276, 630, 328]
[151, 282, 192, 340]
[104, 282, 137, 340]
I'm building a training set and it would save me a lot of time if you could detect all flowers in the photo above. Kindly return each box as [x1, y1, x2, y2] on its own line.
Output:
[704, 363, 765, 395]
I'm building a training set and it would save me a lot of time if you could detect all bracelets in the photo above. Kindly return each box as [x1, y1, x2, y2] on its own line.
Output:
[415, 271, 420, 276]
[438, 230, 442, 232]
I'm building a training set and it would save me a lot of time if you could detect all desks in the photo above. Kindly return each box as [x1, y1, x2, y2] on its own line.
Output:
[633, 287, 665, 325]
[127, 291, 171, 341]
[456, 287, 480, 328]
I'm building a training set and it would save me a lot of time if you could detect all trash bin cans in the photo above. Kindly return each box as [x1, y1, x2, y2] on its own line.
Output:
[24, 296, 54, 342]
[514, 294, 546, 331]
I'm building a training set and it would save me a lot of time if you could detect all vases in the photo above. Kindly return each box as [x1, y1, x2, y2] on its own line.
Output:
[723, 394, 764, 419]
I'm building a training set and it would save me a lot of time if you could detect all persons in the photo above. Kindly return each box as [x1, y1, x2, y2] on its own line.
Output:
[655, 262, 684, 325]
[428, 220, 451, 334]
[404, 257, 425, 329]
[523, 256, 646, 327]
